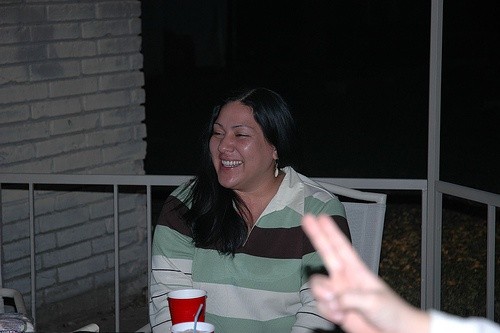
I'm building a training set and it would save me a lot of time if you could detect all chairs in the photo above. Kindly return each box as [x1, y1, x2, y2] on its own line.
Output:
[315, 180, 388, 276]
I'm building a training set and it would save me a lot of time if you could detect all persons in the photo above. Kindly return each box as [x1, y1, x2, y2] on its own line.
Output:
[301, 209, 500, 333]
[149, 79, 354, 333]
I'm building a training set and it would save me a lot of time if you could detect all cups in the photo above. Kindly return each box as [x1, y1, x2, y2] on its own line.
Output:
[166, 289, 207, 324]
[171, 321, 214, 333]
